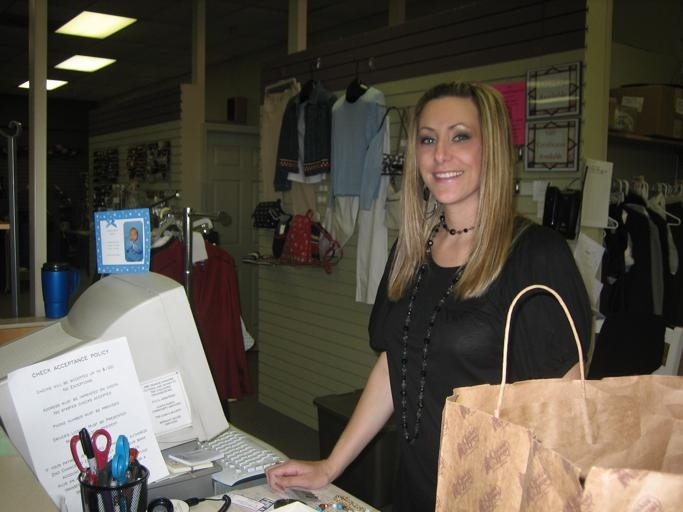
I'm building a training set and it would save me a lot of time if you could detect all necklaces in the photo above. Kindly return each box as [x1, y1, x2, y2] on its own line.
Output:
[436, 210, 485, 237]
[398, 223, 484, 447]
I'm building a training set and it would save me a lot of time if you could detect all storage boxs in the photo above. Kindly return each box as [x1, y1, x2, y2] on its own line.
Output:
[609, 82, 683, 139]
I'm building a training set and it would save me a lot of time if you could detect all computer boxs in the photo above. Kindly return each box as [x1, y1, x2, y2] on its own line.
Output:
[146, 439, 222, 506]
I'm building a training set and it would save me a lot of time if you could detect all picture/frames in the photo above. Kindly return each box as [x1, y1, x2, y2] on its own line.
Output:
[525, 60, 581, 173]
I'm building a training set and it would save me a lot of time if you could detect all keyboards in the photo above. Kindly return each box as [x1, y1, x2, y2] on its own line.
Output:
[201, 428, 281, 487]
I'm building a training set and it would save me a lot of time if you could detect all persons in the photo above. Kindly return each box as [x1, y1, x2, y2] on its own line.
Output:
[122, 226, 143, 265]
[264, 79, 594, 510]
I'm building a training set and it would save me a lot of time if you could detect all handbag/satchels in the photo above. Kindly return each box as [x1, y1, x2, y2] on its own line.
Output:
[434, 280, 683, 512]
[272, 210, 321, 267]
[251, 199, 283, 228]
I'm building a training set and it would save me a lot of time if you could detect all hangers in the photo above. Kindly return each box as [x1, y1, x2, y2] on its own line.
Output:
[602, 174, 683, 230]
[347, 55, 367, 102]
[148, 205, 214, 242]
[299, 59, 320, 102]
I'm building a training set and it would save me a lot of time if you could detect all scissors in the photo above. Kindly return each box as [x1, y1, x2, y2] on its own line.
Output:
[112, 433, 130, 485]
[70, 429, 112, 474]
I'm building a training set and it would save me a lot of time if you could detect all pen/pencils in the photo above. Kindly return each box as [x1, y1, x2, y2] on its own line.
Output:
[79, 428, 143, 512]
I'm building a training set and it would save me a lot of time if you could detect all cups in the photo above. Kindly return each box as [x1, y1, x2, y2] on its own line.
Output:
[40, 262, 81, 320]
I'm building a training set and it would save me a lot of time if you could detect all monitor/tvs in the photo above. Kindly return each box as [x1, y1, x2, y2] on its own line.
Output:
[0, 270, 231, 479]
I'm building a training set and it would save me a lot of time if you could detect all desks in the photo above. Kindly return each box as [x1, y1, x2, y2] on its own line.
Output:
[0, 316, 381, 512]
